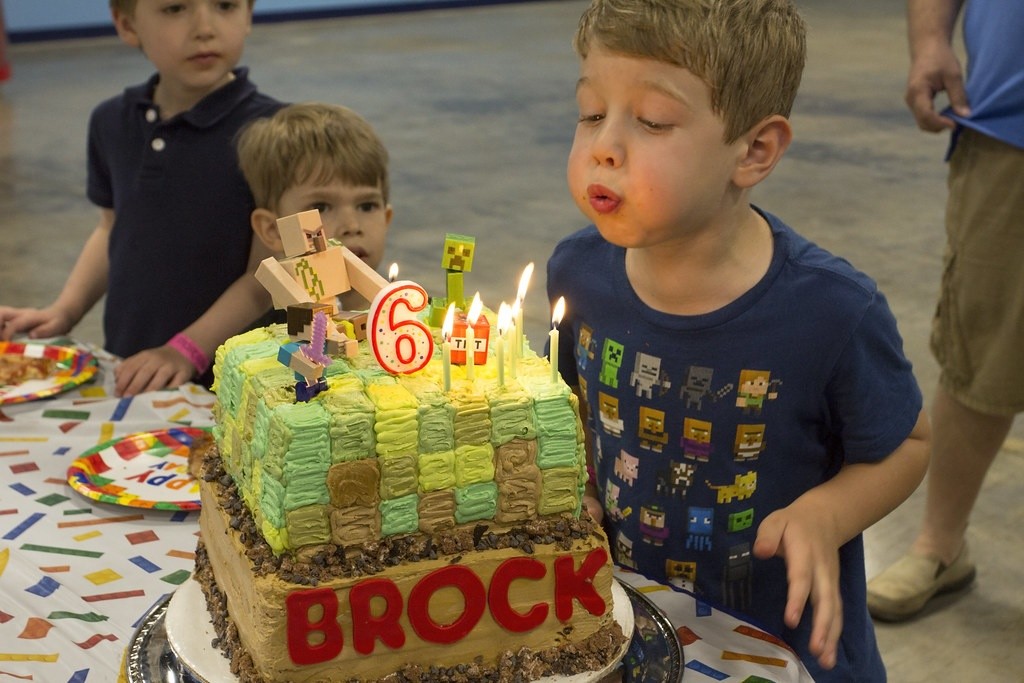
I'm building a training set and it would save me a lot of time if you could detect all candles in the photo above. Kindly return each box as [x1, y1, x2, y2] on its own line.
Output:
[388, 262, 399, 283]
[465, 290, 483, 390]
[509, 298, 520, 380]
[442, 301, 455, 390]
[516, 262, 536, 358]
[496, 301, 505, 385]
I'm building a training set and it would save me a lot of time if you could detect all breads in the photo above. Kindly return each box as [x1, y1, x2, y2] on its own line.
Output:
[0, 353, 56, 386]
[188, 435, 213, 477]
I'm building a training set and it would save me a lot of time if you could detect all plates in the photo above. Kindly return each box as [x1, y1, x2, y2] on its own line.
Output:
[0, 343, 98, 405]
[67, 426, 211, 512]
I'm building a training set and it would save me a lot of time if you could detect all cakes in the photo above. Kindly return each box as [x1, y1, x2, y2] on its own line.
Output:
[195, 295, 630, 683]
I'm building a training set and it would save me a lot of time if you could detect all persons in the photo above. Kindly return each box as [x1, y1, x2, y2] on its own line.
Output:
[236, 101, 392, 311]
[0, 0, 293, 398]
[861, 0, 1024, 622]
[540, 0, 933, 683]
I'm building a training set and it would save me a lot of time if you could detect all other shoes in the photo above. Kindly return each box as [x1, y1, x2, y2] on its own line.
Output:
[866, 521, 977, 622]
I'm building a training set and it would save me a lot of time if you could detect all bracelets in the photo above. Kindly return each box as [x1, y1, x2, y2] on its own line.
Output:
[168, 334, 210, 376]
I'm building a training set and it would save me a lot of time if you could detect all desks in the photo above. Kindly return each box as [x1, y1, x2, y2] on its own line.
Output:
[0, 336, 815, 683]
